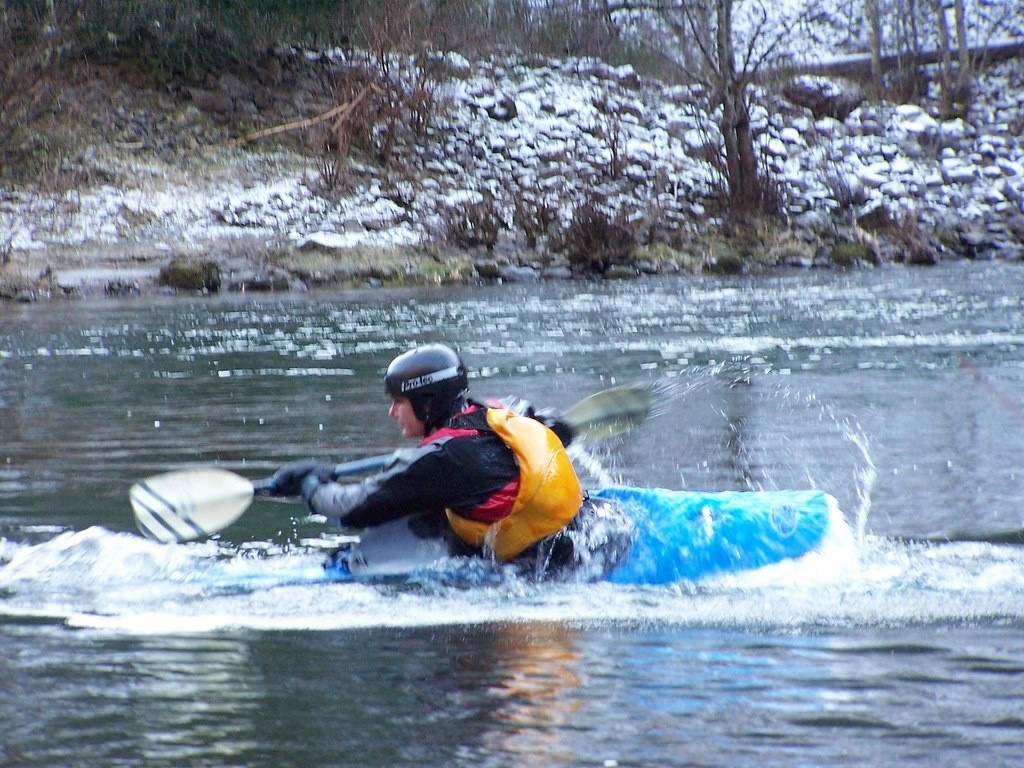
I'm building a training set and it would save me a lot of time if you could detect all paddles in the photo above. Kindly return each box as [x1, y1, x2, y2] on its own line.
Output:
[127, 384, 653, 544]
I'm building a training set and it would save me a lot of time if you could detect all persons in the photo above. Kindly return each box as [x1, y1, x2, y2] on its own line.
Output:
[264, 343, 590, 584]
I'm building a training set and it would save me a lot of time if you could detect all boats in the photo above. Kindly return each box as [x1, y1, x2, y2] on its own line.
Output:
[162, 482, 858, 606]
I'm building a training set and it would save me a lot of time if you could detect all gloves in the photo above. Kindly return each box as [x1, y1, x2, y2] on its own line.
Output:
[268, 460, 334, 515]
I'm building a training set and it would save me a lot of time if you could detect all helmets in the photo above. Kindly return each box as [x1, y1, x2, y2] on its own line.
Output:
[383, 343, 469, 419]
